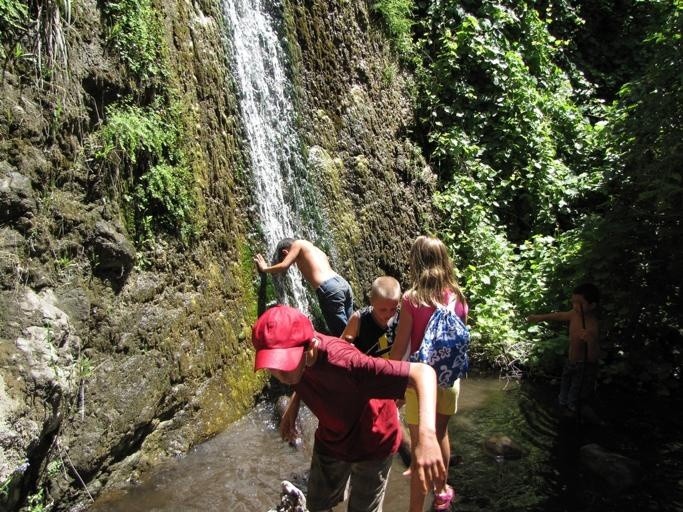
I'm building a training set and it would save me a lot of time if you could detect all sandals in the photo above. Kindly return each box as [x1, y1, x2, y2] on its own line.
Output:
[433, 485, 454, 512]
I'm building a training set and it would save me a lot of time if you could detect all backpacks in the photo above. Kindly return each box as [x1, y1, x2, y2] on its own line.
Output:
[409, 283, 471, 390]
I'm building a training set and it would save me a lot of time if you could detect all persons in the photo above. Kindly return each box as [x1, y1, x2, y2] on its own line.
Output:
[250, 304, 447, 511]
[524, 281, 602, 427]
[388, 235, 468, 511]
[336, 275, 414, 475]
[252, 236, 354, 338]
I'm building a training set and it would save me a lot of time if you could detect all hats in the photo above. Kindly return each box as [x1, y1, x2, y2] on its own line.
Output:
[250, 304, 317, 373]
